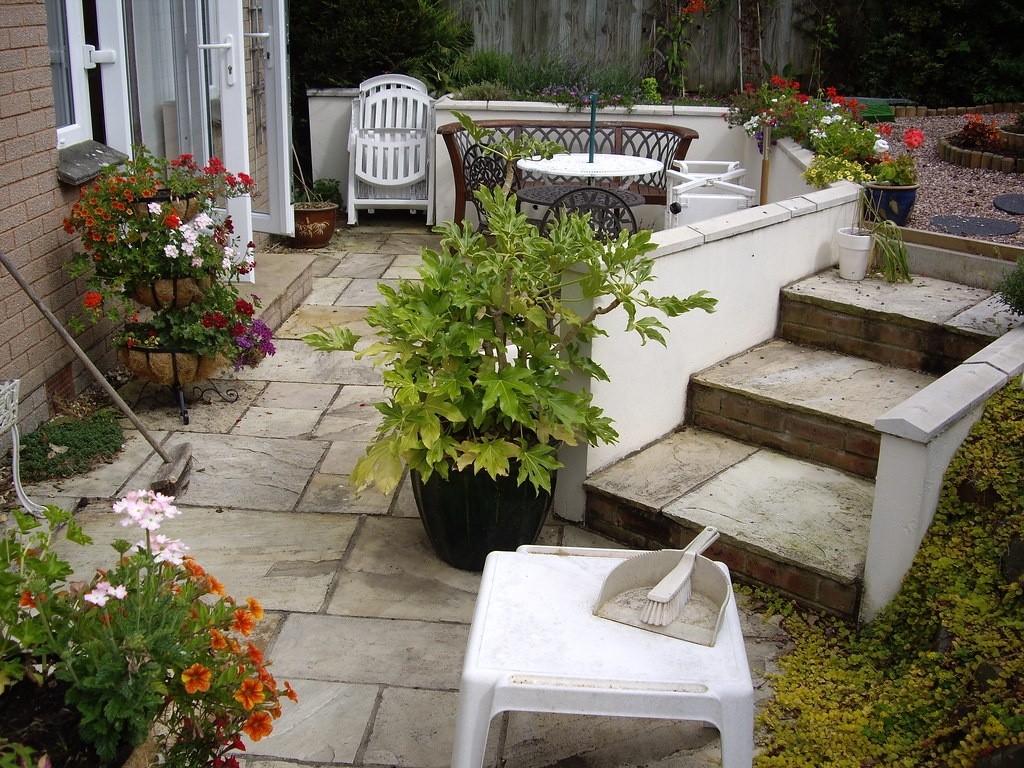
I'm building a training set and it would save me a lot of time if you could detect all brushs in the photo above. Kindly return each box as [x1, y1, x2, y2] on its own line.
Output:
[639, 524, 721, 628]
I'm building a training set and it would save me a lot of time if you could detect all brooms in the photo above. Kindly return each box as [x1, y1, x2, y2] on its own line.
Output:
[0, 240, 196, 498]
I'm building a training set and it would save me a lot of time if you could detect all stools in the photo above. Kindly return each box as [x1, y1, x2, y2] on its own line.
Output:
[446, 539, 758, 768]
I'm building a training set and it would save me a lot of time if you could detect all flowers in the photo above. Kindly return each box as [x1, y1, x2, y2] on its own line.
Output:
[799, 125, 925, 189]
[54, 214, 259, 331]
[61, 141, 263, 249]
[111, 282, 276, 371]
[1, 489, 299, 768]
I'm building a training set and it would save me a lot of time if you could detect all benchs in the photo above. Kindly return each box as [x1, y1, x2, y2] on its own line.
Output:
[437, 118, 701, 259]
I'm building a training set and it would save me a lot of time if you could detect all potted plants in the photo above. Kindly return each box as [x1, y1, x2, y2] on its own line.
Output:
[838, 186, 915, 286]
[300, 108, 721, 572]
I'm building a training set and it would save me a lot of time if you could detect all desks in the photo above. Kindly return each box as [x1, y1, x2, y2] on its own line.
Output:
[515, 181, 645, 240]
[515, 149, 664, 186]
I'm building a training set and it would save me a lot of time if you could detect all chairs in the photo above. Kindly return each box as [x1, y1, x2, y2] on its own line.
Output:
[459, 144, 545, 259]
[347, 74, 434, 228]
[539, 185, 640, 253]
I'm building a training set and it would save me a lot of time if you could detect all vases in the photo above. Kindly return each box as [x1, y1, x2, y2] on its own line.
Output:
[114, 332, 232, 389]
[2, 671, 160, 767]
[287, 200, 338, 248]
[122, 189, 206, 233]
[864, 180, 921, 227]
[121, 273, 221, 311]
